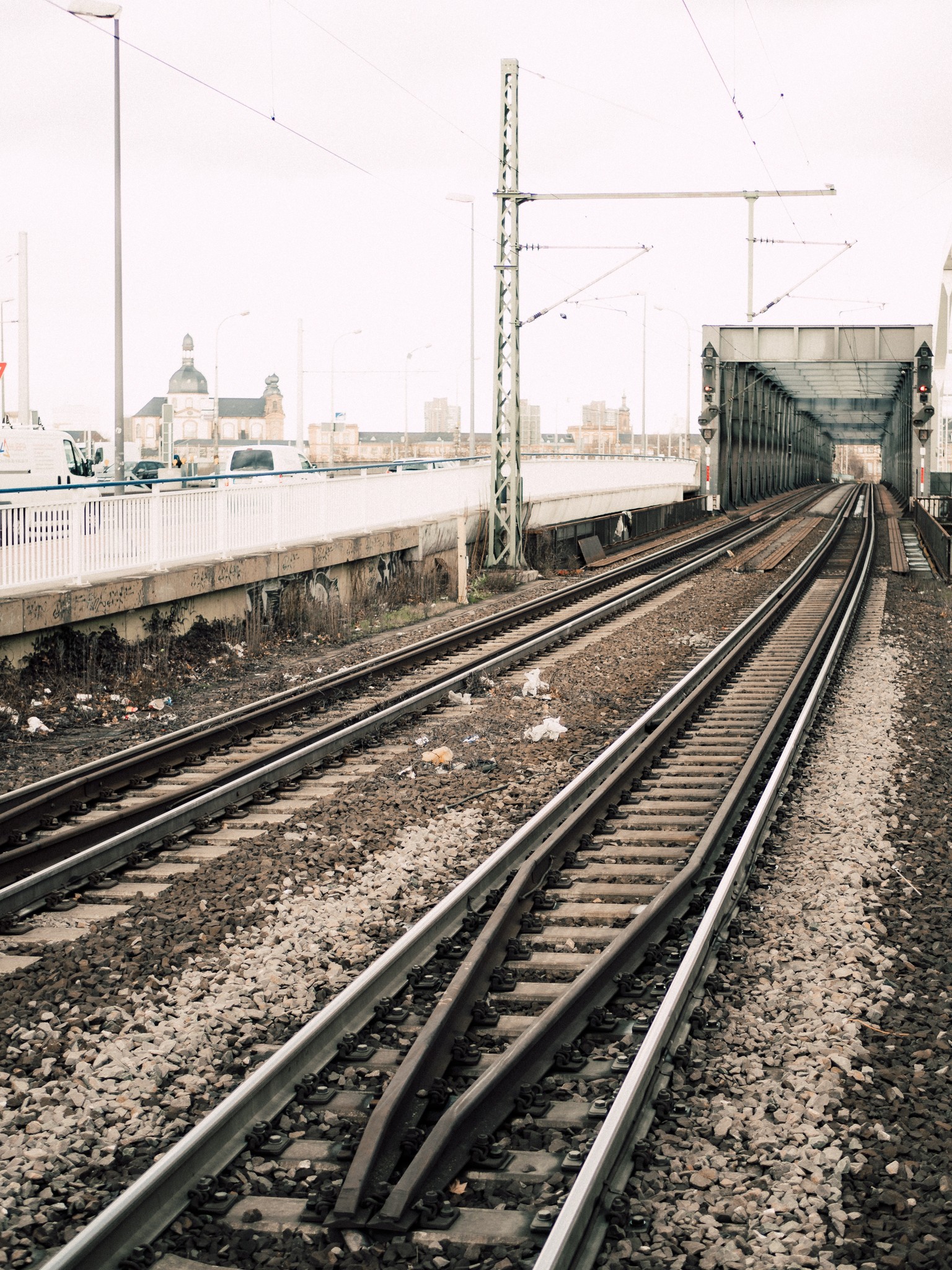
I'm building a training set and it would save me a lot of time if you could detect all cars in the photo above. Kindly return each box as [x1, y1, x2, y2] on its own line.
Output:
[187, 470, 225, 488]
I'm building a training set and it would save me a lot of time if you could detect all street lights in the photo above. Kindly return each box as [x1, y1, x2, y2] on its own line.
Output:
[62, 0, 125, 498]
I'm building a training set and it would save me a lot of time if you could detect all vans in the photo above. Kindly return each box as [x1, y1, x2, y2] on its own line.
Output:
[225, 445, 322, 491]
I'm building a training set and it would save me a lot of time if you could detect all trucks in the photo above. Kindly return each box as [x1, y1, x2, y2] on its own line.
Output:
[0, 428, 101, 538]
[65, 441, 140, 474]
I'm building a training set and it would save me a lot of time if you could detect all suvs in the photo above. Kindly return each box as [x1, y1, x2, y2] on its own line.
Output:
[96, 459, 166, 490]
[385, 457, 455, 474]
